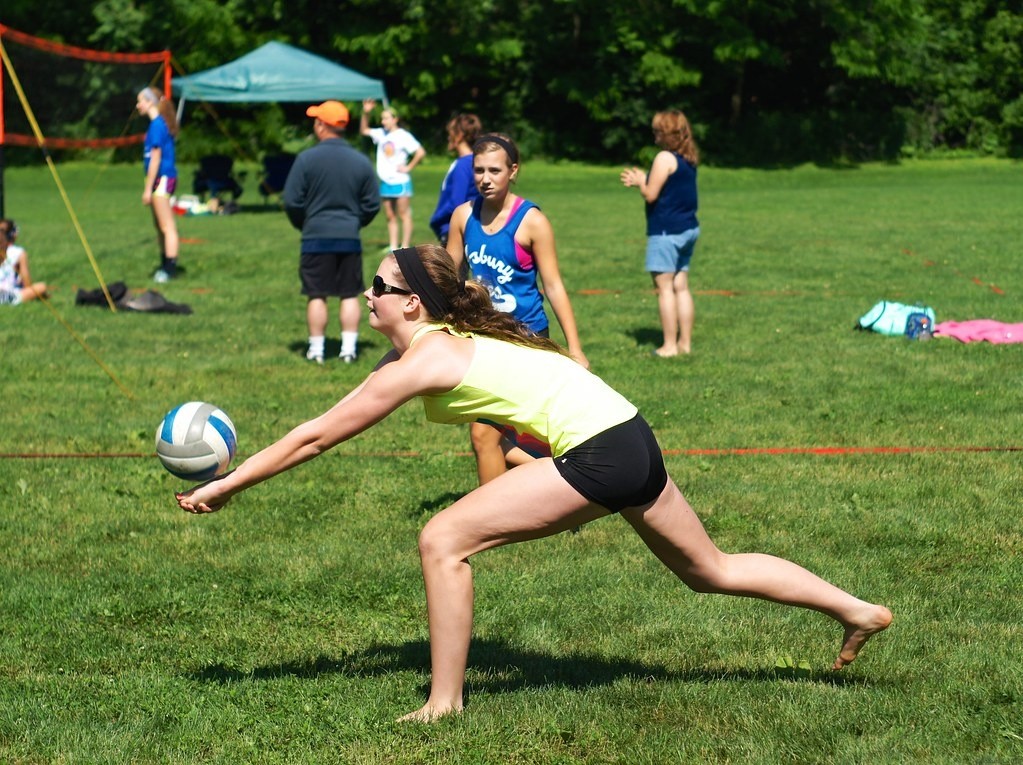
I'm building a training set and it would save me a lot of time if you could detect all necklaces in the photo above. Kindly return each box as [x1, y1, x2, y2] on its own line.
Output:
[488, 227, 495, 232]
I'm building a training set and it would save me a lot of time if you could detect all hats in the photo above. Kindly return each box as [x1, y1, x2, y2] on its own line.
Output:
[306, 100, 350, 128]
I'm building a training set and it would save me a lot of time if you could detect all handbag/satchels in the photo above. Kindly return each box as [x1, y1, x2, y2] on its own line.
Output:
[860, 300, 935, 337]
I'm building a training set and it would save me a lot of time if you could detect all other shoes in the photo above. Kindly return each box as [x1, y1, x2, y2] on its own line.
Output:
[153, 268, 174, 282]
[305, 355, 325, 365]
[339, 354, 359, 364]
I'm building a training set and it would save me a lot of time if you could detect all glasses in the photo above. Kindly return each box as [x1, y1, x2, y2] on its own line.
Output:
[372, 275, 412, 298]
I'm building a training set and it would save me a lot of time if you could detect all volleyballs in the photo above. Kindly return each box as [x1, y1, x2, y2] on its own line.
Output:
[152, 399, 242, 483]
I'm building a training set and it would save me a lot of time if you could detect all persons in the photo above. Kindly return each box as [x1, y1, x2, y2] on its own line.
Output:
[0, 219, 48, 306]
[258, 144, 296, 196]
[445, 134, 590, 370]
[198, 153, 243, 206]
[621, 110, 700, 357]
[428, 114, 482, 249]
[135, 86, 179, 282]
[176, 246, 892, 724]
[360, 97, 427, 251]
[285, 100, 381, 365]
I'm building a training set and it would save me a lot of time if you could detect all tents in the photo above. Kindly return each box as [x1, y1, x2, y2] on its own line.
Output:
[172, 39, 389, 124]
